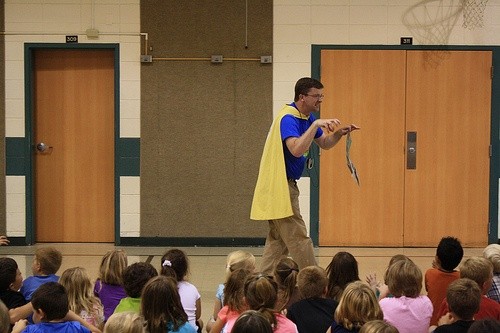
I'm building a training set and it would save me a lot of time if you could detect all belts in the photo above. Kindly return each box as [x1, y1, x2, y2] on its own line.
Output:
[287, 178, 296, 182]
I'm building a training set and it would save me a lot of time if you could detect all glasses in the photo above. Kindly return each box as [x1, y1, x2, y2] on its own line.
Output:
[303, 92, 324, 99]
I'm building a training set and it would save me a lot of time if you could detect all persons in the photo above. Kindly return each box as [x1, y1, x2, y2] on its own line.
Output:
[250, 78, 360, 273]
[0, 237, 500, 333]
